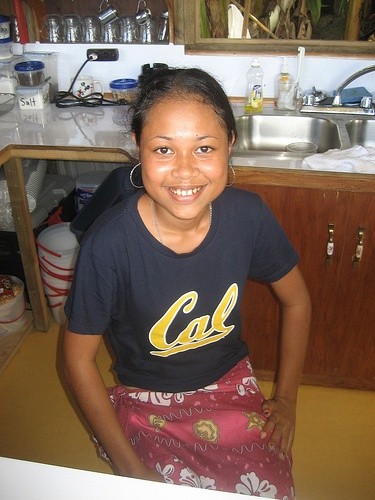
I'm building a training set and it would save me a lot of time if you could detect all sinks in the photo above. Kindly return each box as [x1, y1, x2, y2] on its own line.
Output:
[345, 117, 375, 148]
[230, 114, 342, 154]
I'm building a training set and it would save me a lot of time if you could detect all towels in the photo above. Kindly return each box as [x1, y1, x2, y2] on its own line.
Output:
[302, 145, 375, 174]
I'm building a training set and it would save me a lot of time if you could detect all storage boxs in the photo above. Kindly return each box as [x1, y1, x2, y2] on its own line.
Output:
[0, 174, 81, 299]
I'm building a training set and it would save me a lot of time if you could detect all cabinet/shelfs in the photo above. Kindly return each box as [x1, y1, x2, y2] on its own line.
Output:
[230, 184, 375, 390]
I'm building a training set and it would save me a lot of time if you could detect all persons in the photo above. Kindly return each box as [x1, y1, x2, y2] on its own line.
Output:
[63, 69, 313, 500]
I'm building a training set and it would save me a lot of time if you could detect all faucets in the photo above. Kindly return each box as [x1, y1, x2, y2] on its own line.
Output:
[332, 65, 375, 106]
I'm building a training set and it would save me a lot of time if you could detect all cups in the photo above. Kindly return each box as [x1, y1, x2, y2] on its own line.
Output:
[37, 0, 169, 44]
[22, 159, 49, 213]
[72, 76, 105, 103]
[278, 79, 299, 111]
[286, 142, 319, 170]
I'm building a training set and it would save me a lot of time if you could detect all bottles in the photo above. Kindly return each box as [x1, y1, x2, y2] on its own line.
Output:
[244, 58, 264, 113]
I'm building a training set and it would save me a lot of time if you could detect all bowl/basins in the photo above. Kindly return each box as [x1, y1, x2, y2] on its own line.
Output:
[0, 15, 50, 109]
[76, 171, 109, 210]
[35, 223, 80, 326]
[109, 79, 139, 103]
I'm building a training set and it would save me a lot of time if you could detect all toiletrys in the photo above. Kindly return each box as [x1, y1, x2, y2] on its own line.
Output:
[276, 56, 293, 109]
[245, 59, 264, 114]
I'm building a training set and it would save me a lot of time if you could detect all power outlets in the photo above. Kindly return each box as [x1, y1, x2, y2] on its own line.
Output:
[86, 48, 118, 61]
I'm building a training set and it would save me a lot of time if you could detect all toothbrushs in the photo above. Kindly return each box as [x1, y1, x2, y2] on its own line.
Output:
[287, 47, 306, 95]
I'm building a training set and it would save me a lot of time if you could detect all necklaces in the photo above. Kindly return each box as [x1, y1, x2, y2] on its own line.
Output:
[154, 201, 212, 245]
